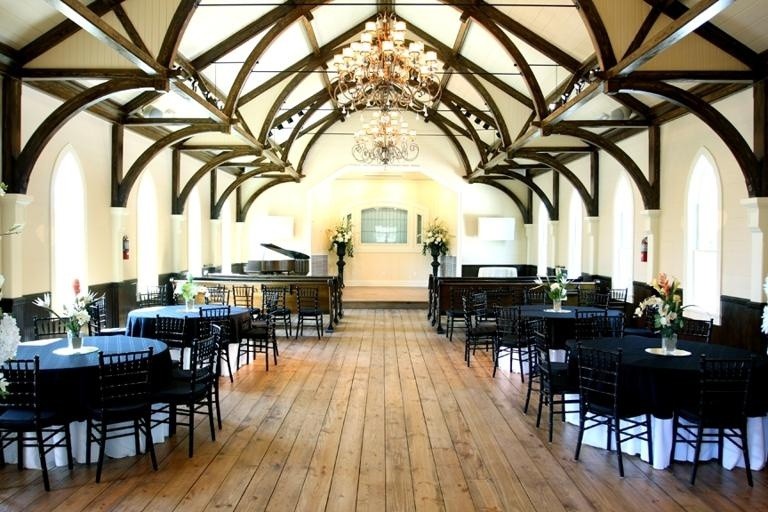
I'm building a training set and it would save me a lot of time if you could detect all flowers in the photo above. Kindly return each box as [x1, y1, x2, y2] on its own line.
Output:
[416, 217, 454, 256]
[327, 213, 354, 258]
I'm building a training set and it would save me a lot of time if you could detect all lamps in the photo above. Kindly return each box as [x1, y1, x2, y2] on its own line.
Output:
[549, 68, 600, 110]
[178, 66, 225, 110]
[329, 6, 445, 117]
[352, 109, 420, 167]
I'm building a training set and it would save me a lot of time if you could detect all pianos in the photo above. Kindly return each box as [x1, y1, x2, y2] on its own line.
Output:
[243, 244, 310, 276]
